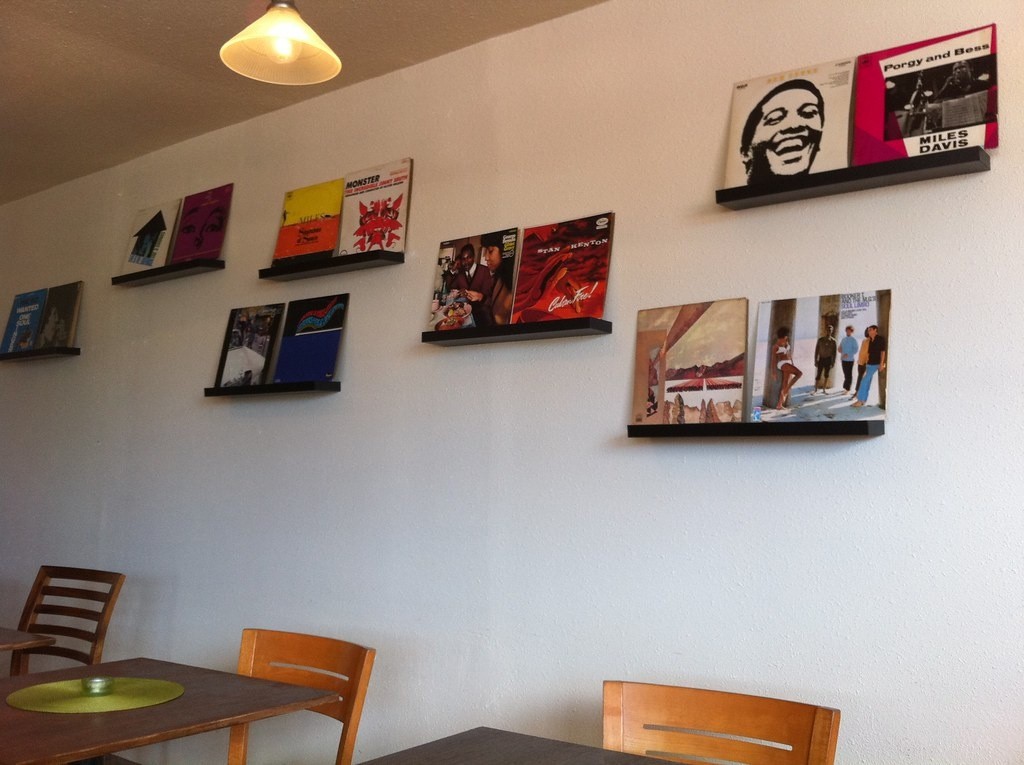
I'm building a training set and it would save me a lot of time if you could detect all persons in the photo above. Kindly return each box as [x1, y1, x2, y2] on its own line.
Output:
[446, 231, 513, 325]
[837, 325, 859, 395]
[937, 60, 990, 96]
[810, 325, 837, 397]
[848, 326, 885, 407]
[39, 303, 69, 348]
[512, 246, 581, 326]
[220, 307, 275, 387]
[772, 326, 803, 410]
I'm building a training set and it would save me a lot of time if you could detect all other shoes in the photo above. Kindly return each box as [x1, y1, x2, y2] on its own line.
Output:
[811, 391, 817, 395]
[852, 390, 857, 398]
[842, 388, 850, 395]
[850, 401, 865, 407]
[777, 406, 786, 410]
[823, 392, 829, 395]
[781, 391, 787, 401]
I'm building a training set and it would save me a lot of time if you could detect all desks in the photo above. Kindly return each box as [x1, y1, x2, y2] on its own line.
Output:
[0, 657, 339, 765]
[356, 726, 689, 765]
[0, 628, 55, 651]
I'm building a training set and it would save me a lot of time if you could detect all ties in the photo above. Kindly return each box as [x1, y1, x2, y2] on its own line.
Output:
[467, 271, 471, 281]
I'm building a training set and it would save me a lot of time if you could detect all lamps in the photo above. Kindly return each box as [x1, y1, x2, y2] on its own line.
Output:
[219, 0, 342, 86]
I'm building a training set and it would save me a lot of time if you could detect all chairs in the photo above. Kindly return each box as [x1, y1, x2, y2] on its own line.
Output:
[228, 628, 377, 765]
[10, 565, 126, 676]
[603, 680, 841, 765]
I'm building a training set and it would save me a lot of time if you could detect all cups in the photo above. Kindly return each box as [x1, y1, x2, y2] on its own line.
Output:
[81, 669, 114, 694]
